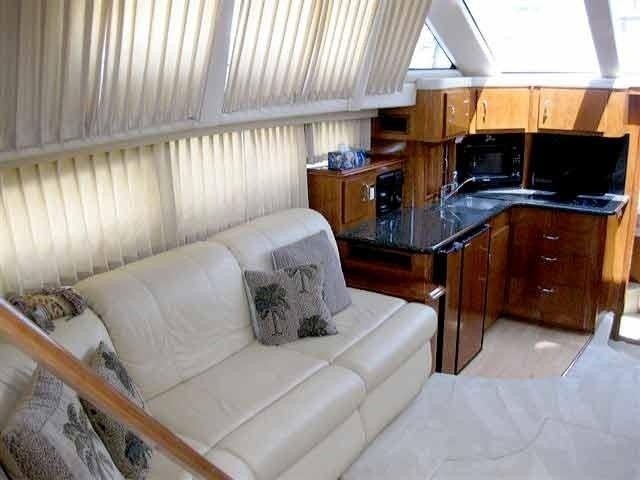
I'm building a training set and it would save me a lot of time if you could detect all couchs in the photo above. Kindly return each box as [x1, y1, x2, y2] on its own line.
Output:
[0, 207, 447, 480]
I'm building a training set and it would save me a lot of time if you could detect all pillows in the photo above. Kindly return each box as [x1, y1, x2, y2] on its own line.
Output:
[0, 339, 156, 480]
[241, 230, 354, 346]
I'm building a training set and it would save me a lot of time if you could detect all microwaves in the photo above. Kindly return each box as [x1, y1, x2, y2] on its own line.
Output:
[460, 144, 522, 187]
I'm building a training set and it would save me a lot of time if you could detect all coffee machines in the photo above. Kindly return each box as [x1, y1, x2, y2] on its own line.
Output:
[376, 169, 404, 218]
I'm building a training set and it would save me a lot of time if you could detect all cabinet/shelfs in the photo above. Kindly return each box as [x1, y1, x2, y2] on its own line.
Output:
[308, 171, 370, 224]
[429, 212, 510, 376]
[471, 87, 629, 138]
[504, 204, 629, 334]
[411, 88, 470, 144]
[408, 140, 457, 204]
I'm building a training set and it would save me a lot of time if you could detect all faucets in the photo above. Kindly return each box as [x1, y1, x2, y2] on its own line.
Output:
[440, 176, 477, 210]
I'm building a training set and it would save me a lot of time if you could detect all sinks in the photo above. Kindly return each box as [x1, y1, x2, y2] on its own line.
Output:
[448, 194, 506, 211]
